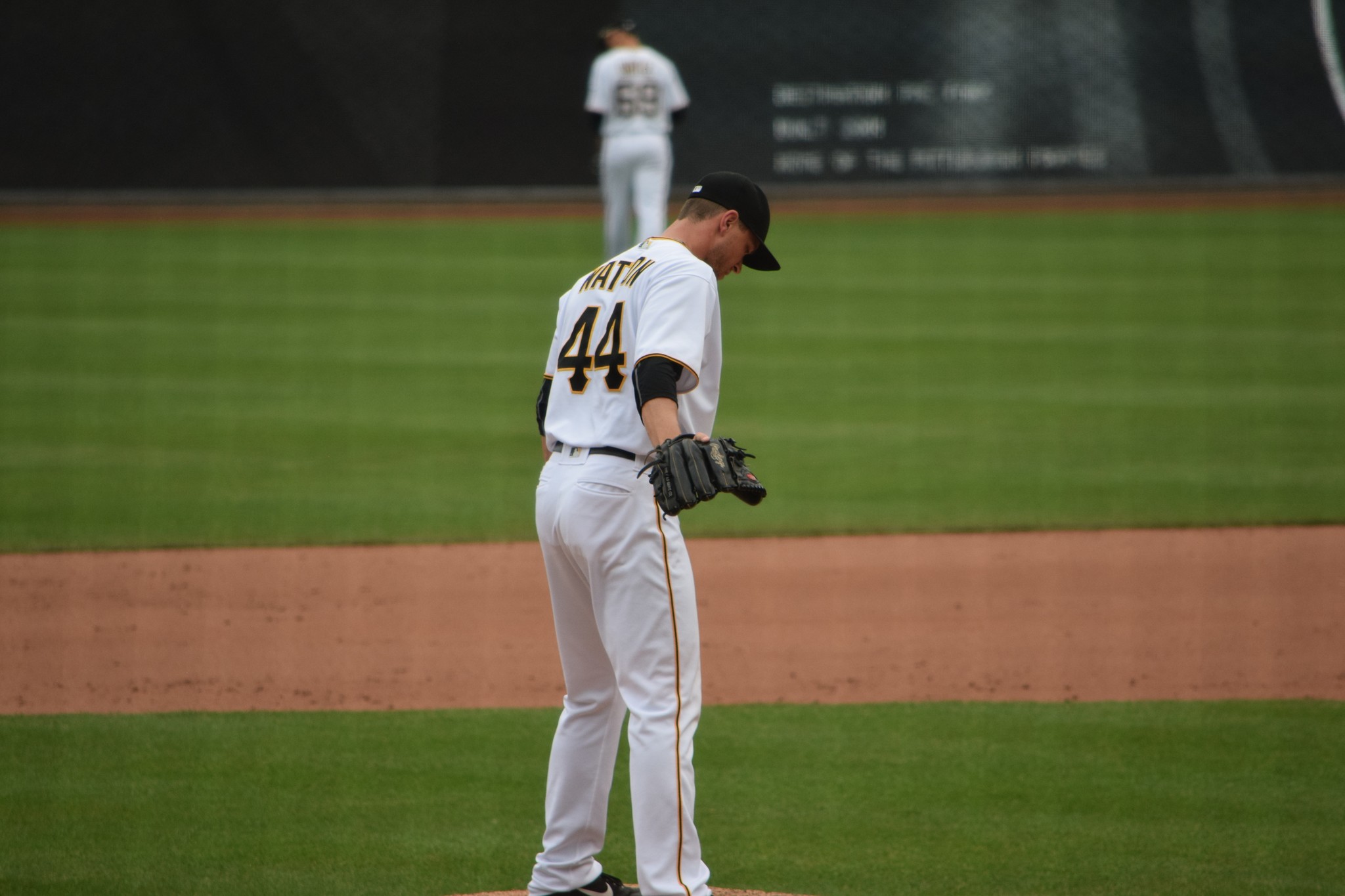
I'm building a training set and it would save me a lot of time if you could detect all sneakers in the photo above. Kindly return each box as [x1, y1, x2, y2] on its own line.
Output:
[548, 870, 641, 896]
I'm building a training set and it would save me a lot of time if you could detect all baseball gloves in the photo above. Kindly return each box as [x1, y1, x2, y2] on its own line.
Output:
[647, 433, 767, 517]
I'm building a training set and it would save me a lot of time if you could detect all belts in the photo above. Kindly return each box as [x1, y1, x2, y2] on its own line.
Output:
[552, 438, 638, 460]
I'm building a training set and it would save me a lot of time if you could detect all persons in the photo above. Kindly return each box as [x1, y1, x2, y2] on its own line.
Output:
[581, 6, 690, 264]
[526, 172, 781, 896]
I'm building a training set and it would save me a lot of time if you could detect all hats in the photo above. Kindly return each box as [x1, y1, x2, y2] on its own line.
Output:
[688, 172, 781, 271]
